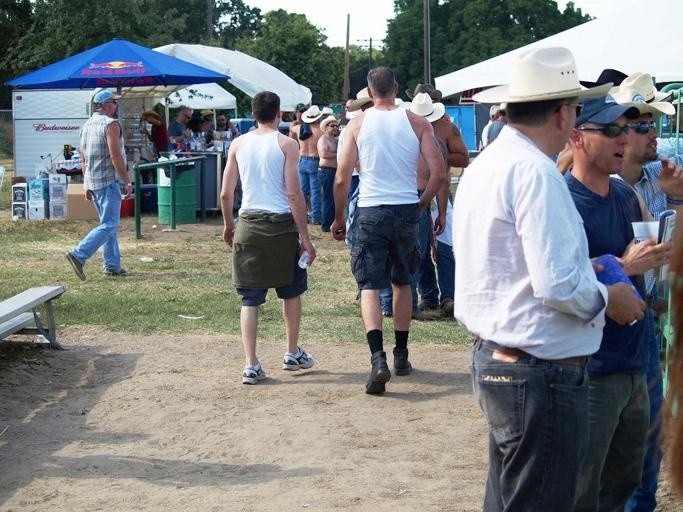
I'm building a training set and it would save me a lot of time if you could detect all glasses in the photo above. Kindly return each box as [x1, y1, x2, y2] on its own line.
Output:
[628, 122, 656, 134]
[581, 124, 632, 139]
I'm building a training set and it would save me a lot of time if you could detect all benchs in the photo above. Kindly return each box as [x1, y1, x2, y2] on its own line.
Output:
[0, 286, 66, 354]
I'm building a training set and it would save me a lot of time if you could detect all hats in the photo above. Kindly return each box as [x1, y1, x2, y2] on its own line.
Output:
[142, 110, 161, 121]
[295, 84, 446, 133]
[94, 90, 122, 104]
[470, 46, 677, 125]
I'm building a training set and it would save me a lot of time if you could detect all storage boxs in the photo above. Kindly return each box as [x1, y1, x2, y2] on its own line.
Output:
[10, 173, 135, 220]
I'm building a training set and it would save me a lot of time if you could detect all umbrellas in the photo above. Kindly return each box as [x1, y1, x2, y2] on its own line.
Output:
[137, 42, 313, 135]
[2, 37, 233, 146]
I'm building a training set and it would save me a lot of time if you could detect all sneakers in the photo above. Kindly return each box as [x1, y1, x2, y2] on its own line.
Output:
[66, 252, 86, 281]
[105, 269, 127, 276]
[366, 299, 454, 395]
[242, 364, 265, 385]
[283, 348, 314, 371]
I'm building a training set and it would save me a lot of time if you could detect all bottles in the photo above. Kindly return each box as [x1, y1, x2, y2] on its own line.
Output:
[296, 242, 316, 271]
[188, 131, 206, 153]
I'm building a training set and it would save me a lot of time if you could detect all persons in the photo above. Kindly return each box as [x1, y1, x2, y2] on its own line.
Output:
[281, 83, 508, 320]
[140, 106, 235, 153]
[66, 91, 132, 282]
[328, 66, 448, 397]
[218, 92, 316, 383]
[452, 45, 682, 512]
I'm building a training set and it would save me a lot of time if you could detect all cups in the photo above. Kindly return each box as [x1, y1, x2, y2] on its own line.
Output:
[630, 220, 661, 248]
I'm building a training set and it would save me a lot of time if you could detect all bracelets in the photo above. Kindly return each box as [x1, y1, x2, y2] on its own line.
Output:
[122, 183, 131, 186]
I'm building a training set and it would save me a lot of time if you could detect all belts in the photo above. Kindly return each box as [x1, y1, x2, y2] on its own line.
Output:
[477, 333, 591, 368]
